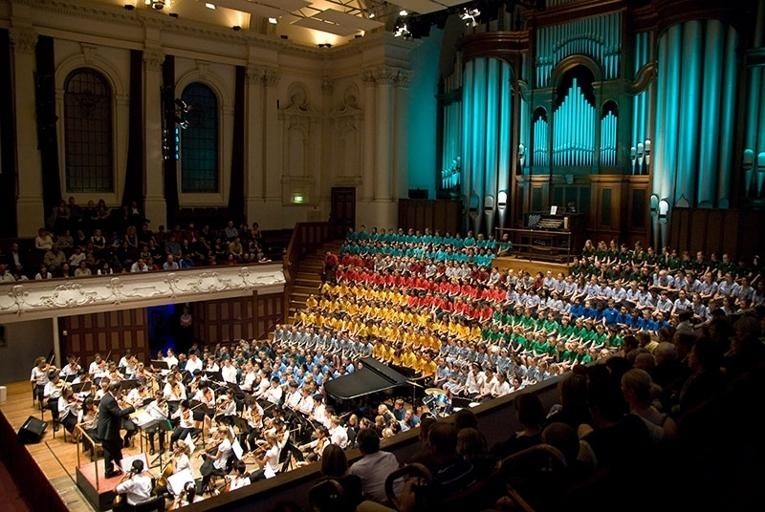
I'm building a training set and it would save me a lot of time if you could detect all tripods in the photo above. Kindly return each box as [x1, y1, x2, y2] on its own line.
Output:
[149, 424, 167, 473]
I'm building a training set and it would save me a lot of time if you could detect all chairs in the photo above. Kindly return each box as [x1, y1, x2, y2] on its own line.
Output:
[193, 412, 206, 446]
[30, 380, 67, 443]
[136, 497, 165, 512]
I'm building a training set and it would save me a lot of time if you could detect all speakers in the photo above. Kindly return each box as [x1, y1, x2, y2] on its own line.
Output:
[17, 415, 48, 444]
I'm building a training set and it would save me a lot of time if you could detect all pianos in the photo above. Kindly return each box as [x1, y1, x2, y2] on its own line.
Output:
[323, 358, 425, 422]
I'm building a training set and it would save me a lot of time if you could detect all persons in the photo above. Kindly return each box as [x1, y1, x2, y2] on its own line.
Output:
[1, 194, 765, 512]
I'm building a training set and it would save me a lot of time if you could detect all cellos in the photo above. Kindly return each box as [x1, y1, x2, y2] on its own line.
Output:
[152, 448, 188, 496]
[112, 467, 140, 508]
[167, 489, 186, 512]
[217, 471, 251, 496]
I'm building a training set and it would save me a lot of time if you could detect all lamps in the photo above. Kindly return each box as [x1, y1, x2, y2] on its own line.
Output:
[124, 4, 362, 48]
[175, 98, 192, 129]
[458, 7, 482, 38]
[392, 12, 421, 42]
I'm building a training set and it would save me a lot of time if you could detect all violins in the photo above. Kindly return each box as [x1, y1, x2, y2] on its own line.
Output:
[310, 439, 325, 454]
[171, 372, 285, 459]
[41, 355, 167, 416]
